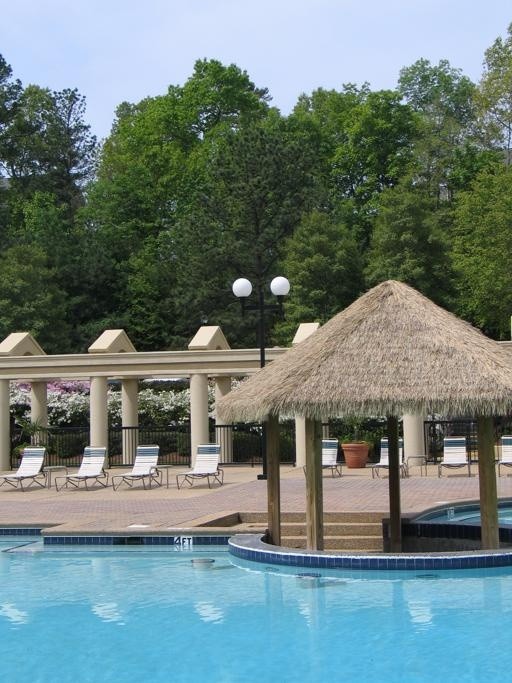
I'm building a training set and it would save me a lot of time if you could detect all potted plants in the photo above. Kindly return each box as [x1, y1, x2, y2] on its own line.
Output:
[337, 414, 380, 468]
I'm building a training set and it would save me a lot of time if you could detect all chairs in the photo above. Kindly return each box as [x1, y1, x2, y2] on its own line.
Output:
[302, 437, 342, 476]
[370, 434, 511, 478]
[1, 443, 225, 489]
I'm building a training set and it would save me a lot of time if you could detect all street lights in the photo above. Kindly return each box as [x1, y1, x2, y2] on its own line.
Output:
[231, 276, 290, 479]
